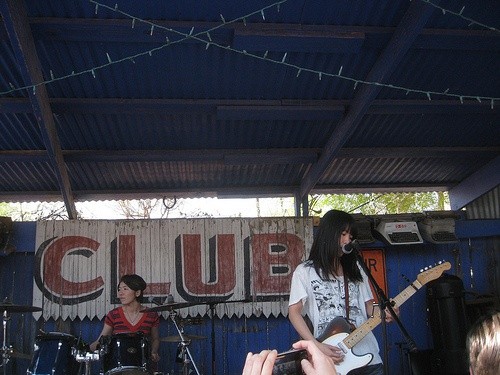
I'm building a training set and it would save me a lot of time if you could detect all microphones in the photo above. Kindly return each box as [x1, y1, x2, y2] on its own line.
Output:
[148, 296, 163, 306]
[342, 239, 359, 255]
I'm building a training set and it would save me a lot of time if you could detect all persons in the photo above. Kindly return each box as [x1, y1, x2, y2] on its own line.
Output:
[288, 209, 399, 375]
[242, 340, 337, 375]
[88, 274, 160, 371]
[464, 310, 500, 375]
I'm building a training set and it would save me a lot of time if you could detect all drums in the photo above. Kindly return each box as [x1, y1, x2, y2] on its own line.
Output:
[99, 361, 153, 375]
[26, 329, 90, 375]
[97, 332, 152, 375]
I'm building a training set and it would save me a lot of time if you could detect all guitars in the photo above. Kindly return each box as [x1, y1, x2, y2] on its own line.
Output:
[315, 259, 452, 375]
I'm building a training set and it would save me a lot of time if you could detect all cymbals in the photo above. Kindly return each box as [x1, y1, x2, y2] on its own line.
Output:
[0, 302, 44, 314]
[139, 302, 203, 312]
[0, 349, 32, 359]
[159, 335, 208, 342]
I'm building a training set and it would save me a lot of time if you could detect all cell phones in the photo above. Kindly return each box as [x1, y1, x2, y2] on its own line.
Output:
[272, 348, 312, 375]
